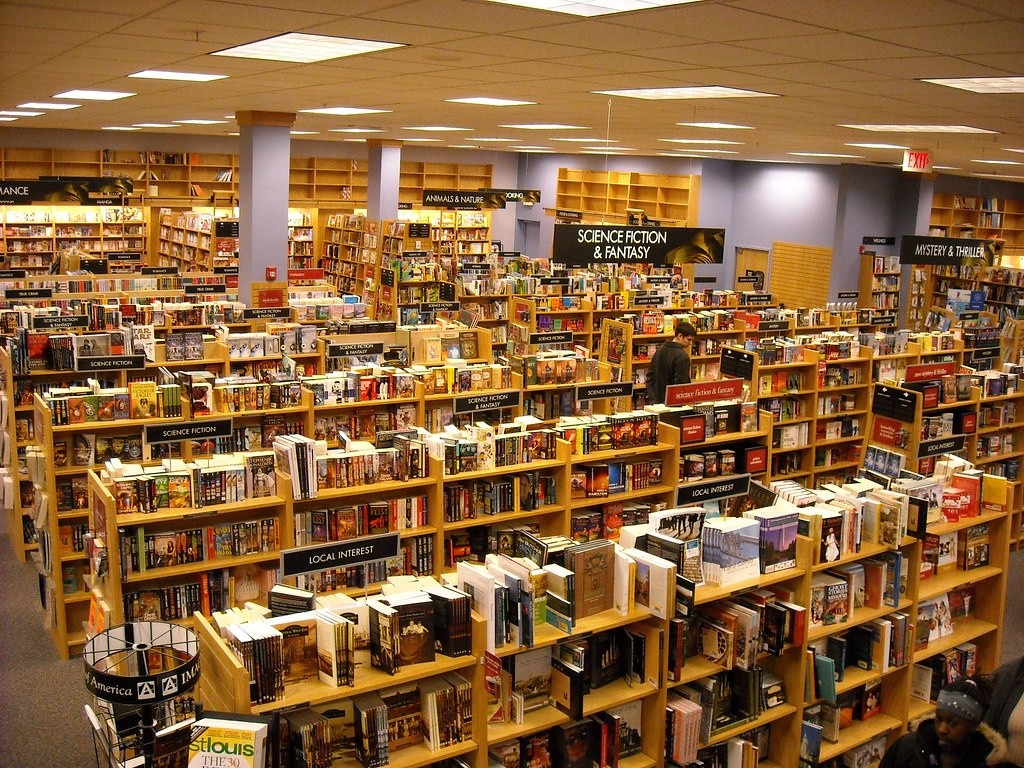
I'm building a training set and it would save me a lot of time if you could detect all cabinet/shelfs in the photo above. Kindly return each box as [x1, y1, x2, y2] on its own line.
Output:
[0, 147, 1024, 768]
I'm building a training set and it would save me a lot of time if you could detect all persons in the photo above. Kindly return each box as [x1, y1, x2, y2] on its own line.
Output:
[645, 321, 696, 404]
[983, 656, 1024, 768]
[877, 672, 1024, 768]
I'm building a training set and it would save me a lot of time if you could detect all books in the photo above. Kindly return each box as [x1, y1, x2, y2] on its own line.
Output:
[0, 195, 1024, 768]
